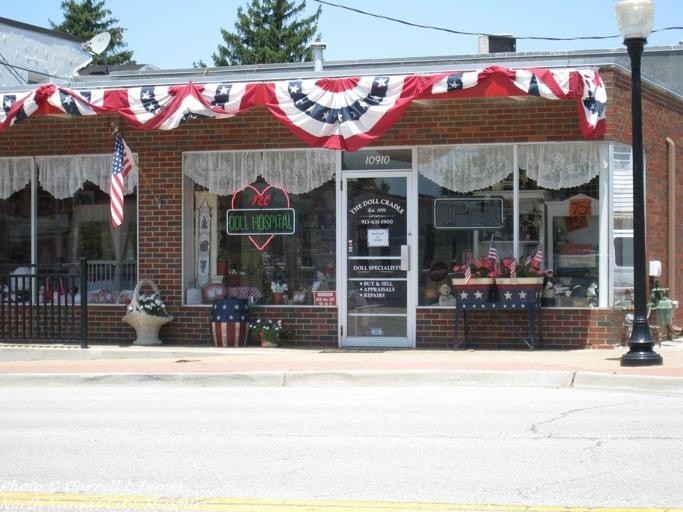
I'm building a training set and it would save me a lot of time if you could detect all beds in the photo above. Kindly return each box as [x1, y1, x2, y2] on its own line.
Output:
[77, 260, 137, 290]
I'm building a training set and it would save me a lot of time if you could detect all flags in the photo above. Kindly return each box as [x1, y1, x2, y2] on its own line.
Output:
[489, 240, 500, 264]
[534, 247, 543, 263]
[509, 259, 517, 277]
[110, 134, 133, 230]
[465, 261, 472, 285]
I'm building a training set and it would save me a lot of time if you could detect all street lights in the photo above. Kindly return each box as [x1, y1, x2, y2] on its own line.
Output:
[613, 1, 662, 366]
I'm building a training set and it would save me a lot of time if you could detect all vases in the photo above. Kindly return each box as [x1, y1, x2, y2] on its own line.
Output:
[272, 293, 282, 304]
[261, 340, 277, 347]
[452, 277, 545, 308]
[121, 278, 175, 346]
[283, 294, 288, 301]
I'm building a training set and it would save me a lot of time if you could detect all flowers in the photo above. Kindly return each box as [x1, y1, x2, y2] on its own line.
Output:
[126, 293, 168, 317]
[247, 316, 288, 345]
[452, 256, 537, 278]
